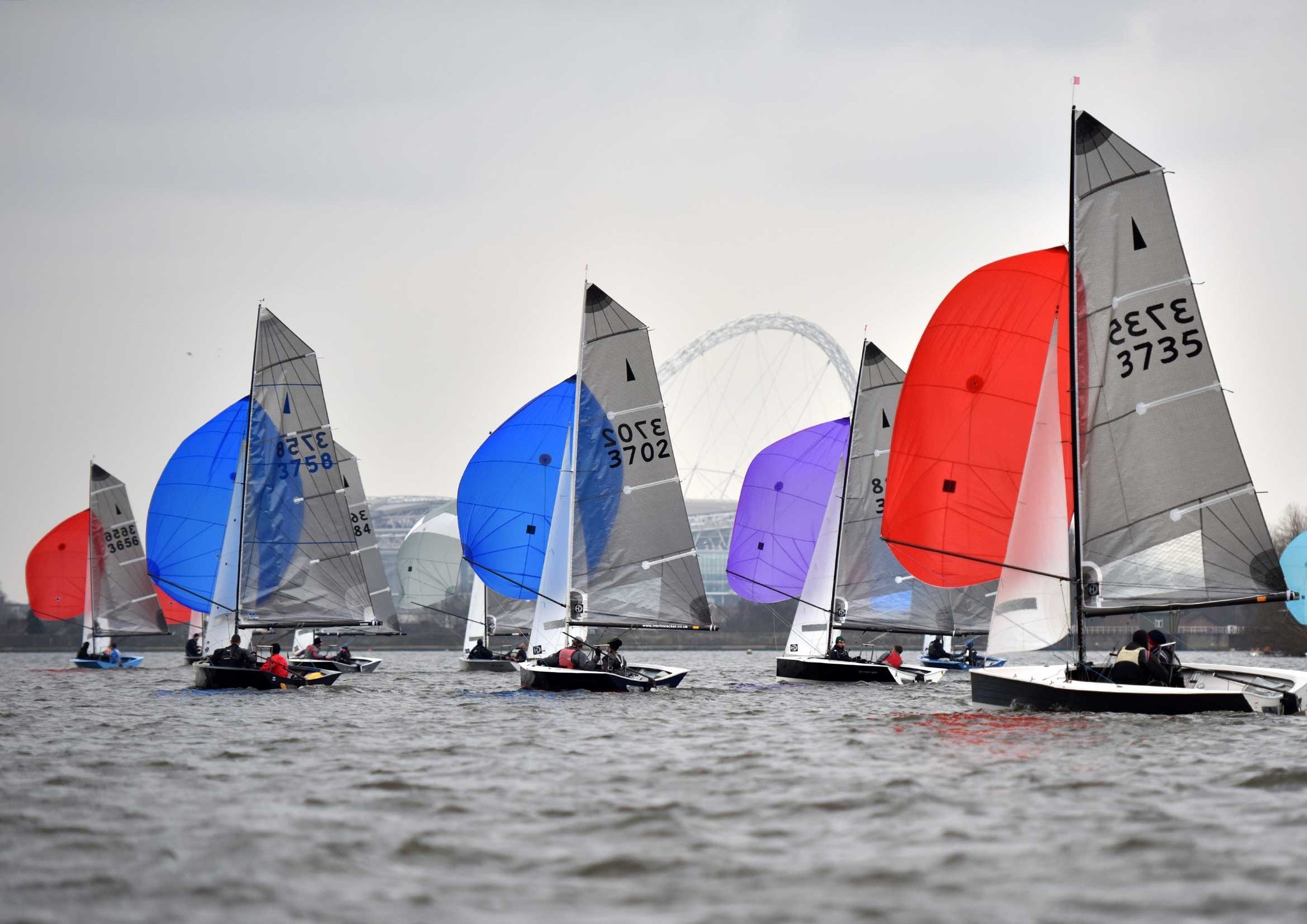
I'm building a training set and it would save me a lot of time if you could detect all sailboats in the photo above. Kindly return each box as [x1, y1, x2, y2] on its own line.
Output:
[455, 262, 723, 694]
[138, 298, 410, 695]
[725, 328, 1010, 682]
[390, 494, 536, 673]
[24, 456, 176, 669]
[873, 100, 1307, 719]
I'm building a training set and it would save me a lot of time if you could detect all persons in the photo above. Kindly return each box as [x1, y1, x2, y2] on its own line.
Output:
[295, 637, 331, 660]
[538, 636, 602, 671]
[602, 638, 623, 671]
[76, 642, 93, 659]
[1111, 629, 1175, 686]
[213, 634, 256, 668]
[103, 643, 121, 664]
[185, 633, 202, 656]
[334, 646, 351, 664]
[494, 642, 527, 662]
[874, 645, 903, 668]
[466, 639, 493, 660]
[259, 643, 288, 678]
[956, 640, 977, 665]
[928, 634, 954, 662]
[831, 636, 867, 663]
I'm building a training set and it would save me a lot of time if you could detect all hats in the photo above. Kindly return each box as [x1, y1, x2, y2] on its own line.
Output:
[342, 646, 347, 649]
[572, 636, 583, 645]
[314, 637, 321, 644]
[966, 642, 974, 647]
[608, 638, 622, 650]
[1147, 630, 1166, 647]
[936, 635, 942, 639]
[836, 636, 844, 643]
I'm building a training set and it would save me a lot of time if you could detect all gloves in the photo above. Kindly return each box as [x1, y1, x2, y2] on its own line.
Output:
[595, 646, 602, 656]
[537, 660, 541, 666]
